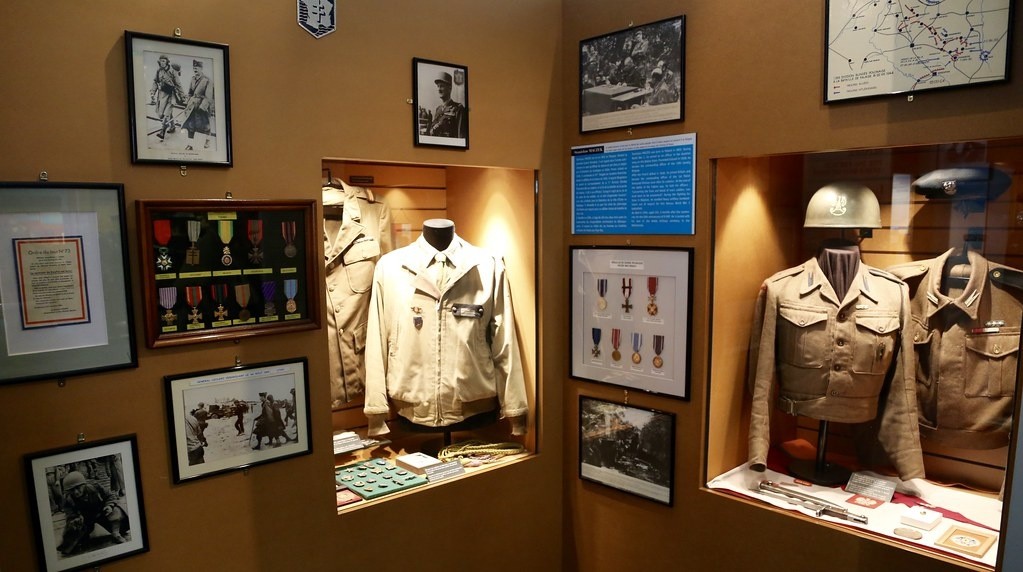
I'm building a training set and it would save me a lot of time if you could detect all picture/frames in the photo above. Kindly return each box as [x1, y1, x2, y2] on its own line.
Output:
[23, 433, 151, 572]
[578, 394, 678, 509]
[568, 245, 695, 403]
[123, 29, 234, 169]
[578, 14, 687, 134]
[0, 182, 139, 386]
[823, 0, 1015, 105]
[163, 356, 314, 487]
[412, 57, 469, 150]
[134, 198, 321, 349]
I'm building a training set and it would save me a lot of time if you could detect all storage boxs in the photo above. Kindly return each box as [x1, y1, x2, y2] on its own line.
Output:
[395, 452, 442, 475]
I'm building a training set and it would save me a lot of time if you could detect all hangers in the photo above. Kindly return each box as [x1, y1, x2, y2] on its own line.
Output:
[322, 168, 343, 189]
[948, 230, 982, 264]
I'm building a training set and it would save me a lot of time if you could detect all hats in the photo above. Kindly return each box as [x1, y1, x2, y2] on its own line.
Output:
[435, 72, 452, 85]
[192, 59, 203, 67]
[259, 392, 267, 396]
[911, 164, 1012, 211]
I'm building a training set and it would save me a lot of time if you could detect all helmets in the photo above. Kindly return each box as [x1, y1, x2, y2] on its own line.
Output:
[159, 55, 170, 63]
[803, 180, 883, 229]
[62, 471, 86, 491]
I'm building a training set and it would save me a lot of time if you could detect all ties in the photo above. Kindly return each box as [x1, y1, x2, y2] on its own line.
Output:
[433, 251, 447, 294]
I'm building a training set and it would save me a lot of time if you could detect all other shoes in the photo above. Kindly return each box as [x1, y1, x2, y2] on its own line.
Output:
[236, 432, 241, 436]
[204, 140, 209, 148]
[273, 443, 281, 448]
[167, 127, 175, 133]
[265, 442, 272, 445]
[252, 445, 260, 450]
[157, 133, 164, 139]
[184, 144, 193, 150]
[285, 438, 291, 443]
[111, 534, 126, 544]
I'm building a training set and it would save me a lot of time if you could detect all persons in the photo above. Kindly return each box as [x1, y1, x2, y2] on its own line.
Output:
[184, 402, 208, 466]
[745, 245, 925, 481]
[582, 30, 678, 109]
[233, 388, 299, 451]
[44, 452, 129, 555]
[364, 218, 530, 438]
[149, 54, 213, 151]
[419, 71, 466, 137]
